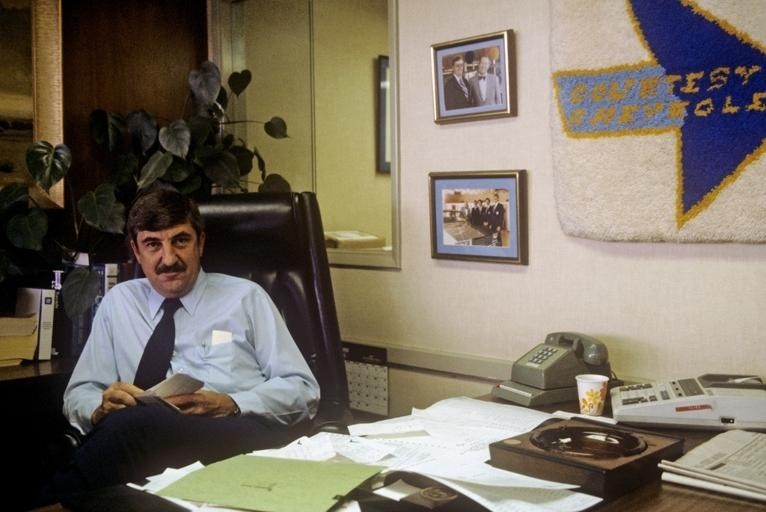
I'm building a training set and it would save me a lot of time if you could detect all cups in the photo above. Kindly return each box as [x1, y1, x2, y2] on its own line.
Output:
[576, 374, 609, 417]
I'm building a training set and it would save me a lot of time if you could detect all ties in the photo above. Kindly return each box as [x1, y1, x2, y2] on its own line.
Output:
[133, 298, 184, 391]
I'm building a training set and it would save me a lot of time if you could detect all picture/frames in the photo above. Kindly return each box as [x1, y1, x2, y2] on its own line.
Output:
[428, 169, 528, 266]
[375, 53, 392, 175]
[430, 28, 517, 126]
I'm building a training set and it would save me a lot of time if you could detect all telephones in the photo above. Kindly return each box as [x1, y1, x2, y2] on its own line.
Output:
[489, 332, 625, 408]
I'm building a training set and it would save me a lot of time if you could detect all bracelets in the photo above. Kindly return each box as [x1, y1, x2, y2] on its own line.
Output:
[232, 409, 242, 415]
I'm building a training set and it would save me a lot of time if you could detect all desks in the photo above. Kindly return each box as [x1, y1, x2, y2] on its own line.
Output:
[0, 359, 70, 393]
[19, 395, 766, 512]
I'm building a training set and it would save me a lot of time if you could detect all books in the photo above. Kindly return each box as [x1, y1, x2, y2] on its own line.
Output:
[0, 252, 144, 368]
[324, 230, 384, 249]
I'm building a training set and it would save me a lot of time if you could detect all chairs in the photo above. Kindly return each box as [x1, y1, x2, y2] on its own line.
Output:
[128, 190, 349, 434]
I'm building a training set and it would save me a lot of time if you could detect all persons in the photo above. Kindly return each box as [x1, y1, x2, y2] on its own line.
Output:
[449, 193, 504, 247]
[445, 56, 471, 109]
[52, 190, 321, 511]
[470, 56, 501, 106]
